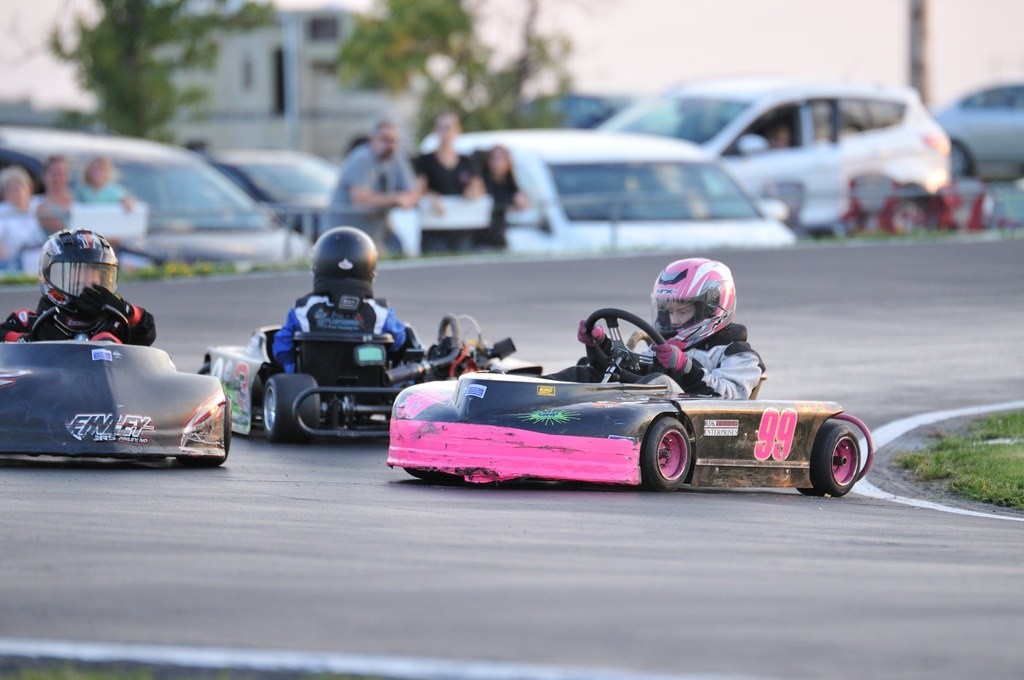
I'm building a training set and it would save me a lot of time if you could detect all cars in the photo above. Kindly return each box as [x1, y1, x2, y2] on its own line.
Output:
[0, 125, 311, 271]
[932, 80, 1024, 185]
[520, 92, 633, 130]
[597, 77, 951, 241]
[168, 145, 344, 246]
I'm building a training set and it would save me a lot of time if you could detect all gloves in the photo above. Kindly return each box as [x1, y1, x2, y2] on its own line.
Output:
[17, 334, 35, 343]
[578, 320, 605, 346]
[651, 344, 686, 373]
[76, 284, 131, 321]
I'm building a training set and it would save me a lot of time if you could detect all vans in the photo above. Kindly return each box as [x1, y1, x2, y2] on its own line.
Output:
[384, 130, 796, 258]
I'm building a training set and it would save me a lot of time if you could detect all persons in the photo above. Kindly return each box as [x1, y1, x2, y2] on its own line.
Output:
[0, 225, 157, 348]
[766, 127, 792, 150]
[0, 152, 140, 278]
[320, 112, 534, 261]
[270, 225, 422, 374]
[577, 257, 765, 399]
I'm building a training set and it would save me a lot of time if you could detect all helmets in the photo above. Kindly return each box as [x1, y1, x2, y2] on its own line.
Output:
[310, 225, 379, 298]
[650, 258, 737, 350]
[39, 227, 120, 316]
